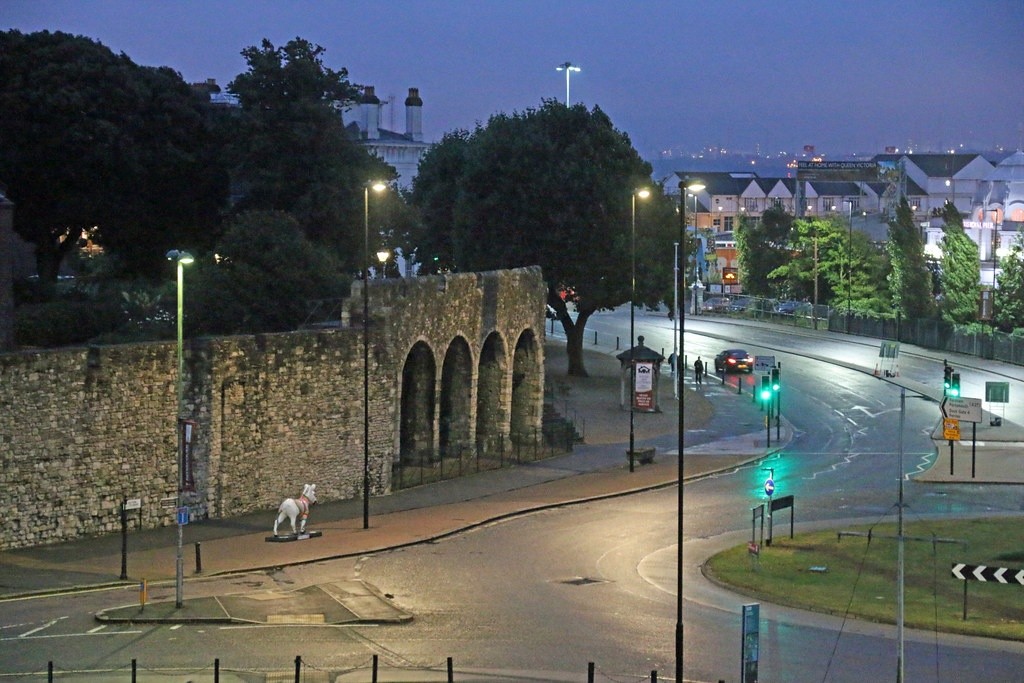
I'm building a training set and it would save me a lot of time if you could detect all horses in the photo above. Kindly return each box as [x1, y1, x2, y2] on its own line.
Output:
[273, 483, 319, 537]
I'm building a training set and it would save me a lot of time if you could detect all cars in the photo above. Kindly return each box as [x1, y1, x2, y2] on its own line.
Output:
[698, 295, 832, 329]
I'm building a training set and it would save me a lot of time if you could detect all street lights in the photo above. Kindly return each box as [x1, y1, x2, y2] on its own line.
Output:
[173, 249, 196, 609]
[556, 61, 581, 109]
[981, 209, 999, 361]
[627, 187, 650, 473]
[688, 193, 698, 316]
[362, 179, 383, 529]
[841, 198, 850, 335]
[672, 174, 707, 682]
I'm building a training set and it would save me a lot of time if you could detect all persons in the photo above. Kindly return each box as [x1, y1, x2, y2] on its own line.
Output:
[694, 356, 703, 385]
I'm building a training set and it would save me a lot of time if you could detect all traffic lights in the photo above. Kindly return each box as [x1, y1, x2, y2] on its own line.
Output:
[943, 366, 961, 398]
[760, 368, 781, 401]
